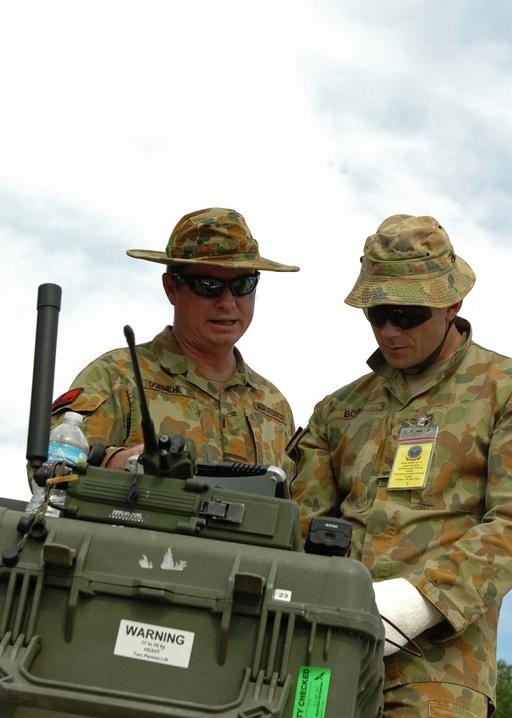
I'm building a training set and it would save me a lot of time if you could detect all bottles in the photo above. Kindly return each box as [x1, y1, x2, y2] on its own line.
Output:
[22, 410, 91, 520]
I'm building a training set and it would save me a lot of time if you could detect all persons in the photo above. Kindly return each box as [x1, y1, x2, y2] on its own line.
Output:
[285, 214, 510, 717]
[45, 206, 296, 480]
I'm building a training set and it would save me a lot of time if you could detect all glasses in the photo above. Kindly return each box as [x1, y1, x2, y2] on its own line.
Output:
[171, 272, 260, 301]
[362, 305, 434, 331]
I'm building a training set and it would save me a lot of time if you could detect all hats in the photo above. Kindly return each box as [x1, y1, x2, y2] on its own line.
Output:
[124, 207, 299, 274]
[343, 213, 476, 310]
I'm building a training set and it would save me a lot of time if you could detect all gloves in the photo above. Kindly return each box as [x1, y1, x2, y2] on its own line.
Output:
[373, 577, 443, 659]
